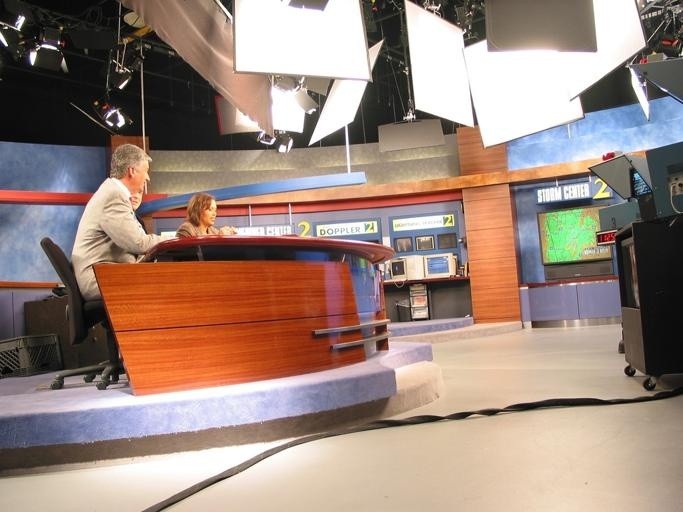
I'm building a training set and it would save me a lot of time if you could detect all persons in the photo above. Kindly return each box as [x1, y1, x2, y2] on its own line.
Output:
[128, 173, 149, 236]
[175, 192, 239, 240]
[69, 143, 180, 302]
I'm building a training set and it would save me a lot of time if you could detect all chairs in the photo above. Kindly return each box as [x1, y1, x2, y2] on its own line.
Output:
[40, 236, 129, 391]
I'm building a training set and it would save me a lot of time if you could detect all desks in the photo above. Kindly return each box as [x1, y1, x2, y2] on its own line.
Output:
[379, 276, 469, 322]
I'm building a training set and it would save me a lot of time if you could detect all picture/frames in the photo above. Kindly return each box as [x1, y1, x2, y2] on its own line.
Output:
[437, 233, 457, 249]
[394, 237, 412, 252]
[415, 235, 435, 251]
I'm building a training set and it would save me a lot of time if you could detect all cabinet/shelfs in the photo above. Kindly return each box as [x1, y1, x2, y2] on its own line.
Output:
[409, 284, 430, 320]
[615, 214, 682, 390]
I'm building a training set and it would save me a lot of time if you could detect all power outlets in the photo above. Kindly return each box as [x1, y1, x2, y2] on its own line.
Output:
[666, 172, 683, 197]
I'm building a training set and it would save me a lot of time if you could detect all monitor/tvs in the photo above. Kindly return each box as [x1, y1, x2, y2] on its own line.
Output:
[536, 204, 614, 265]
[390, 260, 405, 279]
[423, 253, 454, 278]
[629, 166, 652, 197]
